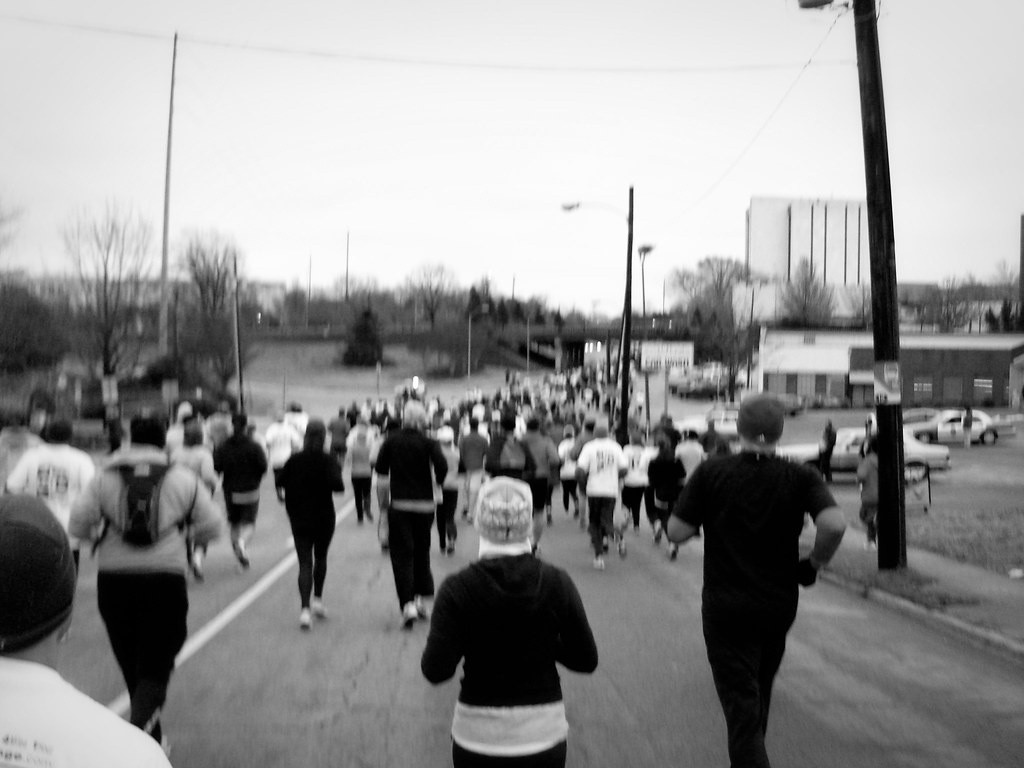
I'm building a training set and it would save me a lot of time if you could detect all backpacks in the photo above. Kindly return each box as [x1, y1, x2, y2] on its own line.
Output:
[116, 465, 170, 547]
[500, 433, 526, 469]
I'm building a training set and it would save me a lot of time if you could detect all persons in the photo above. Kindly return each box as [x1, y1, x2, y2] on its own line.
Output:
[0, 364, 976, 582]
[420, 475, 598, 768]
[280, 420, 344, 628]
[374, 399, 451, 630]
[666, 392, 849, 766]
[69, 403, 224, 746]
[0, 488, 179, 768]
[4, 415, 97, 640]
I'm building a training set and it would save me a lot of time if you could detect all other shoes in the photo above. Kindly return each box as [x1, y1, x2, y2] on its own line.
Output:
[300, 596, 329, 634]
[400, 594, 435, 633]
[233, 539, 250, 569]
[564, 507, 679, 570]
[862, 541, 877, 552]
[546, 513, 552, 525]
[440, 538, 455, 554]
[192, 554, 204, 582]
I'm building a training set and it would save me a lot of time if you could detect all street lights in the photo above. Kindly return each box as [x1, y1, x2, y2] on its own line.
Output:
[799, 0, 906, 572]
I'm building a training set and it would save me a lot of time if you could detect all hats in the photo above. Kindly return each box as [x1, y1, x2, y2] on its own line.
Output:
[474, 475, 534, 541]
[404, 400, 426, 429]
[631, 431, 641, 444]
[564, 424, 574, 438]
[184, 424, 203, 446]
[736, 394, 783, 444]
[593, 426, 607, 438]
[304, 421, 326, 442]
[1, 492, 75, 656]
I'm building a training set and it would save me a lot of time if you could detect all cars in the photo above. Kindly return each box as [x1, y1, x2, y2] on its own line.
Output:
[779, 426, 950, 484]
[865, 407, 936, 425]
[907, 409, 999, 447]
